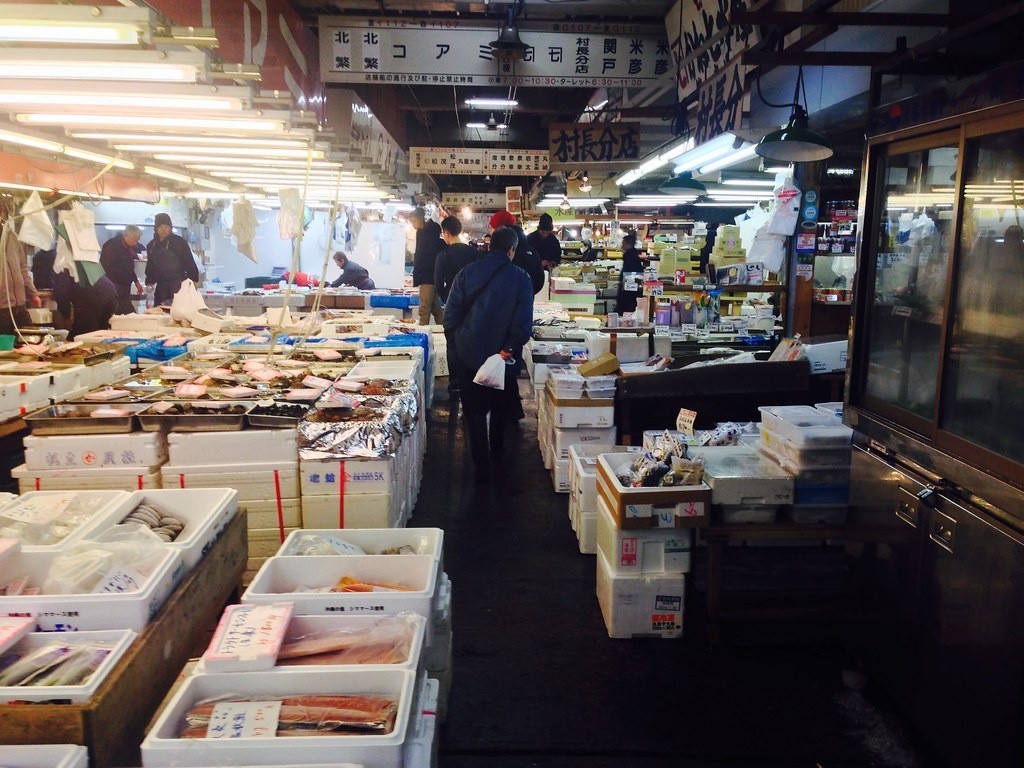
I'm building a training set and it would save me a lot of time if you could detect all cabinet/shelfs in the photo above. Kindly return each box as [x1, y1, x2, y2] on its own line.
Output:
[561, 214, 695, 243]
[842, 96, 1024, 509]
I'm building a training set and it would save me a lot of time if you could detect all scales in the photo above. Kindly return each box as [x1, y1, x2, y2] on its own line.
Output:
[13, 327, 69, 350]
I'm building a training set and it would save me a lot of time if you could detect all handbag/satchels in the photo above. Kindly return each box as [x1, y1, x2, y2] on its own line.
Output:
[169, 278, 209, 323]
[473, 354, 517, 391]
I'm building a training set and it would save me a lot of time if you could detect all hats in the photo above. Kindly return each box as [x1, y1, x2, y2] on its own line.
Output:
[489, 210, 516, 231]
[538, 214, 553, 231]
[410, 207, 425, 220]
[155, 213, 172, 231]
[583, 239, 592, 246]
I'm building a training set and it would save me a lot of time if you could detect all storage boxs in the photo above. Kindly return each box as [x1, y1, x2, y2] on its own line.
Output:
[521, 227, 856, 638]
[0, 284, 454, 768]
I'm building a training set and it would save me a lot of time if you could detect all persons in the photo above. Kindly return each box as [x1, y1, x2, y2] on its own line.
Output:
[0, 201, 206, 344]
[411, 209, 648, 500]
[326, 251, 375, 289]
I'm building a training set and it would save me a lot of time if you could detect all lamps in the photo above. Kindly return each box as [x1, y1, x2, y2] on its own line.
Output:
[0, 0, 419, 222]
[464, 0, 837, 207]
